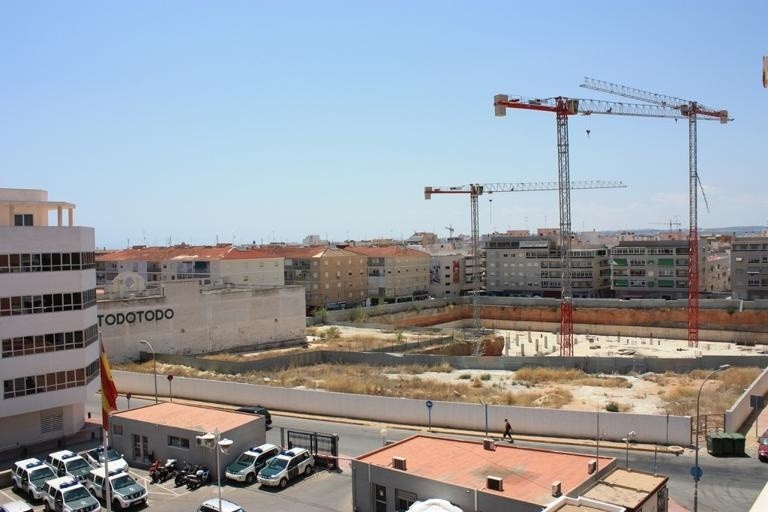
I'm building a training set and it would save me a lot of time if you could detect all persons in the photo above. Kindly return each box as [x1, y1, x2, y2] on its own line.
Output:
[501, 419, 513, 441]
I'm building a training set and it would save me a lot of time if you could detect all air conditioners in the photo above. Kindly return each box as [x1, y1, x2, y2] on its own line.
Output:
[587, 460, 596, 474]
[483, 439, 495, 451]
[391, 456, 407, 470]
[551, 481, 563, 497]
[486, 475, 504, 491]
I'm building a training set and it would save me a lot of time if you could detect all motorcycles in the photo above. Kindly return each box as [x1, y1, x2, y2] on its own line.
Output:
[149, 457, 209, 490]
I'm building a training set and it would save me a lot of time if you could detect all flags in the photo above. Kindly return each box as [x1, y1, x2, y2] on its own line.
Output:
[99, 341, 119, 432]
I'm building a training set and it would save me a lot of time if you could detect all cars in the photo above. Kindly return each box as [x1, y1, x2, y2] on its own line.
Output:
[196, 497, 246, 512]
[235, 407, 272, 429]
[758, 428, 768, 460]
[224, 442, 315, 488]
[0, 445, 147, 512]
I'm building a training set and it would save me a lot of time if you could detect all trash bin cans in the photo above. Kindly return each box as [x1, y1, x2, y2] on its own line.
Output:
[705, 431, 746, 457]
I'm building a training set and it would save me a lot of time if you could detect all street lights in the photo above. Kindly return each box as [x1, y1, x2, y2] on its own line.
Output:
[694, 364, 731, 510]
[140, 340, 158, 405]
[626, 431, 636, 467]
[196, 427, 232, 512]
